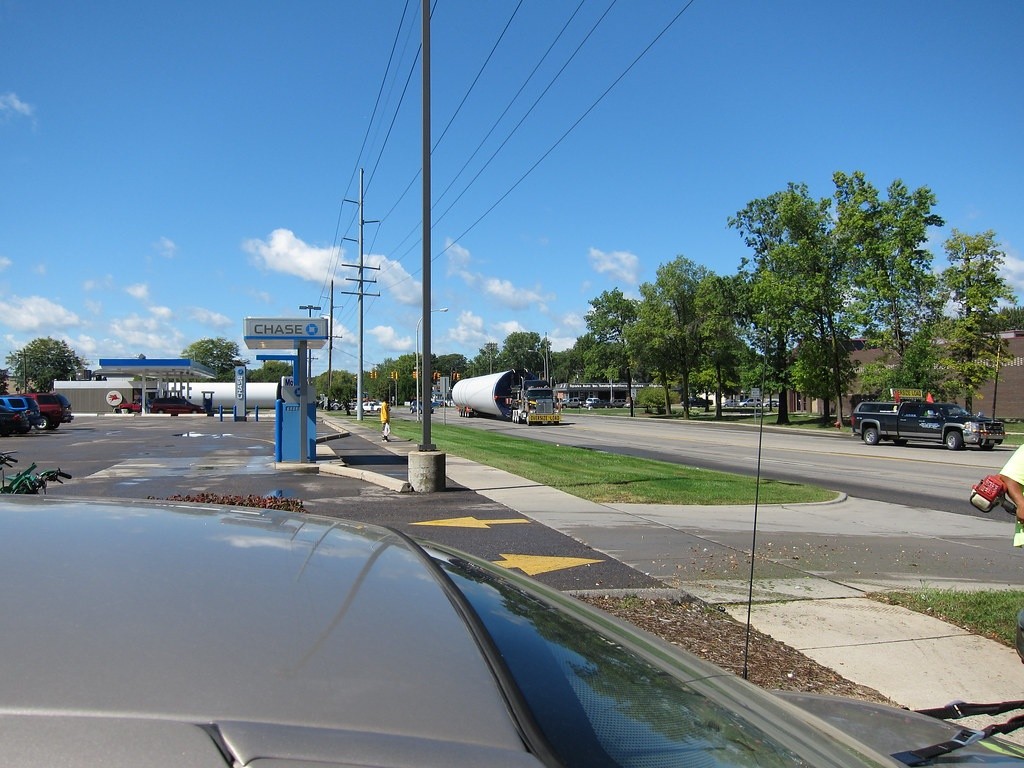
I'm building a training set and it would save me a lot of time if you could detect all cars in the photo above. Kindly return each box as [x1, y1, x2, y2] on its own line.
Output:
[1, 485, 1024, 768]
[0, 404, 24, 436]
[316, 394, 391, 413]
[721, 396, 780, 408]
[409, 400, 434, 415]
[117, 394, 207, 414]
[554, 396, 631, 409]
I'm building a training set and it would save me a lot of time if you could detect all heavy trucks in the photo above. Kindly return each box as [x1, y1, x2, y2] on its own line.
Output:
[451, 368, 565, 426]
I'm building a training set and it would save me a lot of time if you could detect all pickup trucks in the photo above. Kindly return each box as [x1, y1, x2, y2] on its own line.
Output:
[850, 387, 1005, 451]
[680, 397, 714, 408]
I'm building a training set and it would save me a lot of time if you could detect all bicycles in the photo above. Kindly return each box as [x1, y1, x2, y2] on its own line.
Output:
[0, 449, 72, 494]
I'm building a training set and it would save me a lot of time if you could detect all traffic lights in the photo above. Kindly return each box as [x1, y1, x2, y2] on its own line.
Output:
[412, 371, 416, 379]
[452, 373, 456, 381]
[374, 371, 378, 379]
[369, 371, 374, 379]
[395, 371, 400, 379]
[390, 371, 395, 378]
[456, 373, 461, 382]
[436, 373, 441, 380]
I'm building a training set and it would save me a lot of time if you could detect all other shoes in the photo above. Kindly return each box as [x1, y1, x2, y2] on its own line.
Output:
[382, 439, 390, 443]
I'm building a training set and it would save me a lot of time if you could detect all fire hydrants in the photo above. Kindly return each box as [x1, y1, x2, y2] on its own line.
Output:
[833, 420, 842, 429]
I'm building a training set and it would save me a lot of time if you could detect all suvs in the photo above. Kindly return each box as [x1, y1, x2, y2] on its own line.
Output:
[12, 391, 74, 430]
[0, 393, 44, 433]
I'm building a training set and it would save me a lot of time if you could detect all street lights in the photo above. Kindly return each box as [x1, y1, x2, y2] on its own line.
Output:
[299, 304, 322, 385]
[415, 308, 449, 422]
[527, 348, 546, 381]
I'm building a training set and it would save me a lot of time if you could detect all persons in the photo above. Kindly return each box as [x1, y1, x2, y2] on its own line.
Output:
[380, 401, 391, 442]
[146, 399, 154, 414]
[999, 443, 1024, 662]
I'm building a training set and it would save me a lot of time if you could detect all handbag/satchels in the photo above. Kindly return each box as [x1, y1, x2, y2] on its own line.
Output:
[384, 423, 391, 437]
